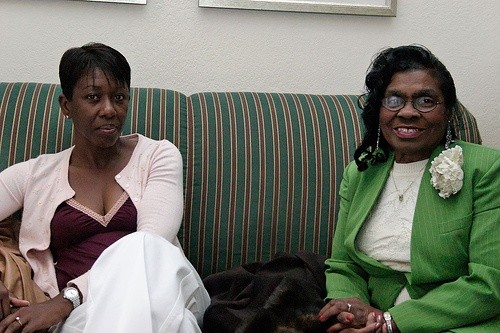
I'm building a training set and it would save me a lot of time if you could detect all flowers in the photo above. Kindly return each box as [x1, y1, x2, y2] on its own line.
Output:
[429, 144, 464, 199]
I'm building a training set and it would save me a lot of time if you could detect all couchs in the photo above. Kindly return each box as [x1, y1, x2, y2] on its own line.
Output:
[0, 83, 482, 278]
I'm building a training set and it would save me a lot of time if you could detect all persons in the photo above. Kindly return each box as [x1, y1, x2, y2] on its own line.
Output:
[0, 40, 212, 333]
[315, 42, 500, 333]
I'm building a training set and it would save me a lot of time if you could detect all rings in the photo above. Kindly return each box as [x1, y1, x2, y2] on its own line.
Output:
[348, 303, 351, 312]
[15, 316, 23, 327]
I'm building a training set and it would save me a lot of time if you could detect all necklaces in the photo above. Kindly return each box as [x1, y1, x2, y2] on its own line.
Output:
[391, 165, 423, 201]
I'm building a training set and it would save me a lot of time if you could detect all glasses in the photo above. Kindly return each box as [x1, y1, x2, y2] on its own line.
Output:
[380, 95, 445, 113]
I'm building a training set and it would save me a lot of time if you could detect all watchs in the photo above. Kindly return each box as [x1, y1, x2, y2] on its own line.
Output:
[61, 286, 80, 309]
[383, 312, 392, 333]
